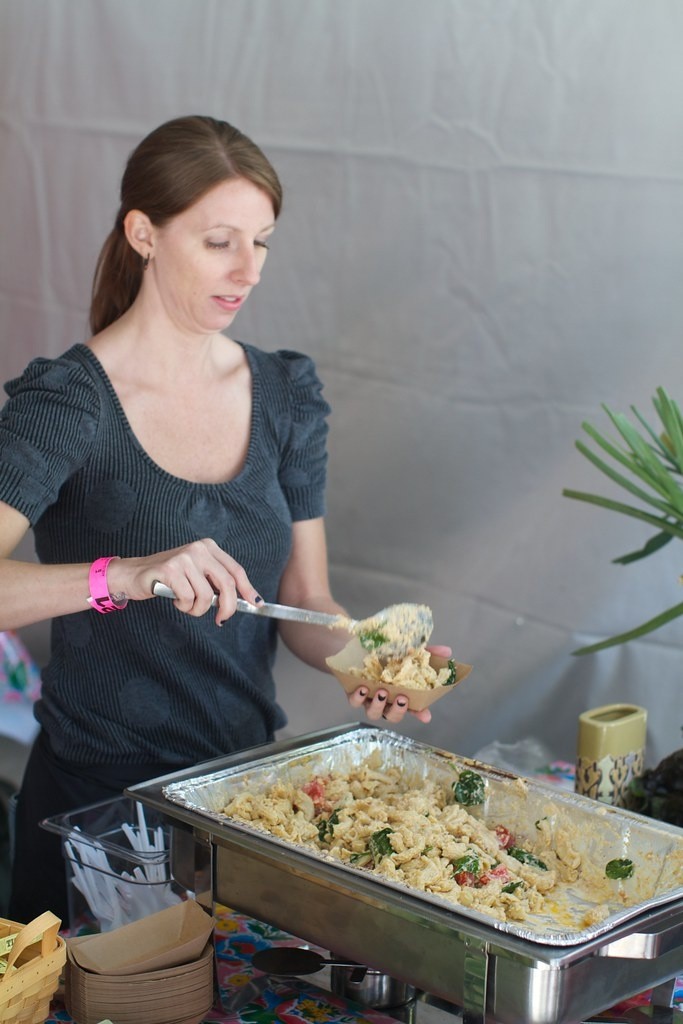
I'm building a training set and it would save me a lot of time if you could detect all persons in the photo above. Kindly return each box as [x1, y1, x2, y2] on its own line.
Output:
[0, 116, 453, 929]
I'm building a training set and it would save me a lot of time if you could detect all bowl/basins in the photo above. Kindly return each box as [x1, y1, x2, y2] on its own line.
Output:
[64, 898, 216, 1024]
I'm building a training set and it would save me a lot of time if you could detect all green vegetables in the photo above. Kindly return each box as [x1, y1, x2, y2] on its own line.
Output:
[359, 619, 456, 686]
[313, 759, 634, 895]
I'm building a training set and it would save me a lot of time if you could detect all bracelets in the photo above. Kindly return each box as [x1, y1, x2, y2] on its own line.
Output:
[86, 555, 128, 615]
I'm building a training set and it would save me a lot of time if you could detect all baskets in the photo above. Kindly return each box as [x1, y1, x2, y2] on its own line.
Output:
[0, 911, 67, 1024]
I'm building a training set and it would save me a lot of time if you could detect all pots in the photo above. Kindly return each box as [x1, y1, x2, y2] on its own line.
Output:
[329, 951, 415, 1010]
[252, 947, 368, 974]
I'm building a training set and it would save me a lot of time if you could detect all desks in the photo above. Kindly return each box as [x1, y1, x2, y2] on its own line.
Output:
[46, 760, 683, 1024]
